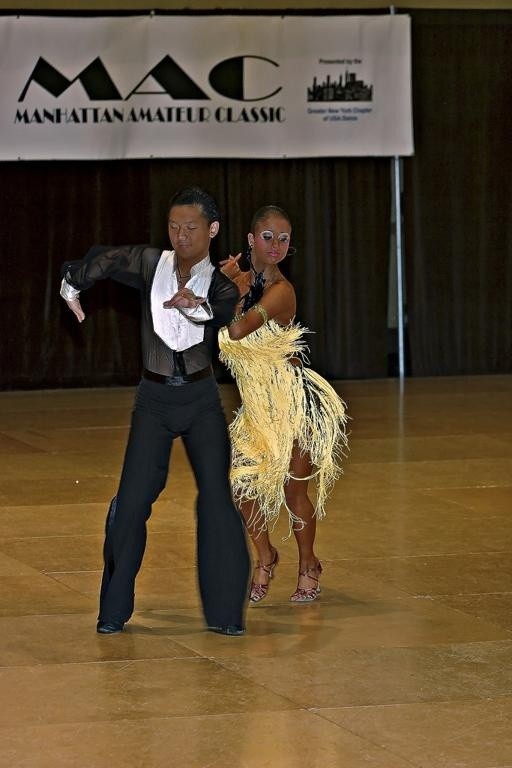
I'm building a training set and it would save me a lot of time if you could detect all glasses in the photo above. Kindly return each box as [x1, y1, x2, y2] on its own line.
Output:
[254, 231, 291, 244]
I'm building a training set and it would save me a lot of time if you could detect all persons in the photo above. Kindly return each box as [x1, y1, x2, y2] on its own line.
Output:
[60, 186, 254, 636]
[216, 206, 350, 604]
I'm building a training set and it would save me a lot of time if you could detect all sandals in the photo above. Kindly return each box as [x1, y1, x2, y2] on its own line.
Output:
[249, 546, 279, 602]
[288, 558, 323, 602]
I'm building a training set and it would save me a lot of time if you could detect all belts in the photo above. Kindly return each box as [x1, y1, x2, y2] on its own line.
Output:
[141, 367, 213, 386]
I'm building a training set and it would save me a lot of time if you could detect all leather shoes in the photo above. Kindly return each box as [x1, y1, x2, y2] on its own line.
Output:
[96, 619, 124, 633]
[206, 622, 246, 635]
